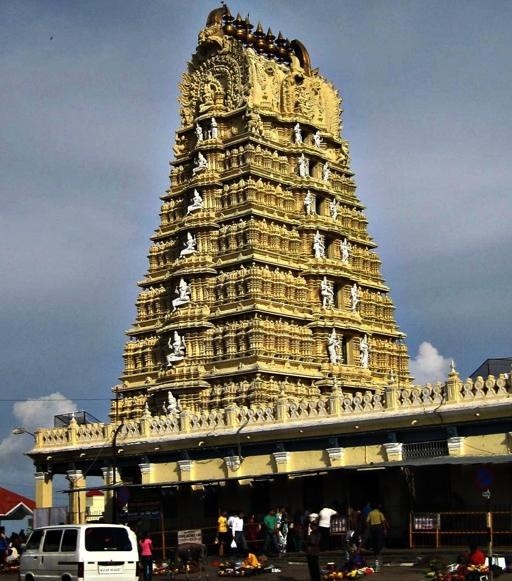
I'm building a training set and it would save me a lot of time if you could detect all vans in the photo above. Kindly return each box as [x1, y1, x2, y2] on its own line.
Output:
[18, 524, 140, 581]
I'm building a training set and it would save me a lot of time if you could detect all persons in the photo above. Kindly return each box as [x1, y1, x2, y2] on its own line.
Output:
[455, 544, 485, 566]
[163, 116, 219, 414]
[216, 503, 390, 581]
[1, 526, 26, 569]
[139, 532, 155, 581]
[293, 122, 369, 369]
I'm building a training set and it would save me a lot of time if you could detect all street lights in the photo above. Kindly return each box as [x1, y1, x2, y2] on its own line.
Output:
[9, 425, 36, 441]
